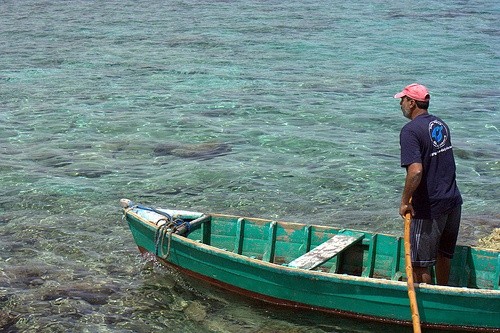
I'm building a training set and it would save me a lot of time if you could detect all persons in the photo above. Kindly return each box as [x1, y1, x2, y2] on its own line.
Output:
[394, 84, 462, 286]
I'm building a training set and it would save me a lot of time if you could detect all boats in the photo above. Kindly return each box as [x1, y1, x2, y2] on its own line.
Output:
[120, 198, 500, 332]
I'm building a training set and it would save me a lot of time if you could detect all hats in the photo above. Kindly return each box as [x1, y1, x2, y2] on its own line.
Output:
[394, 82, 430, 102]
